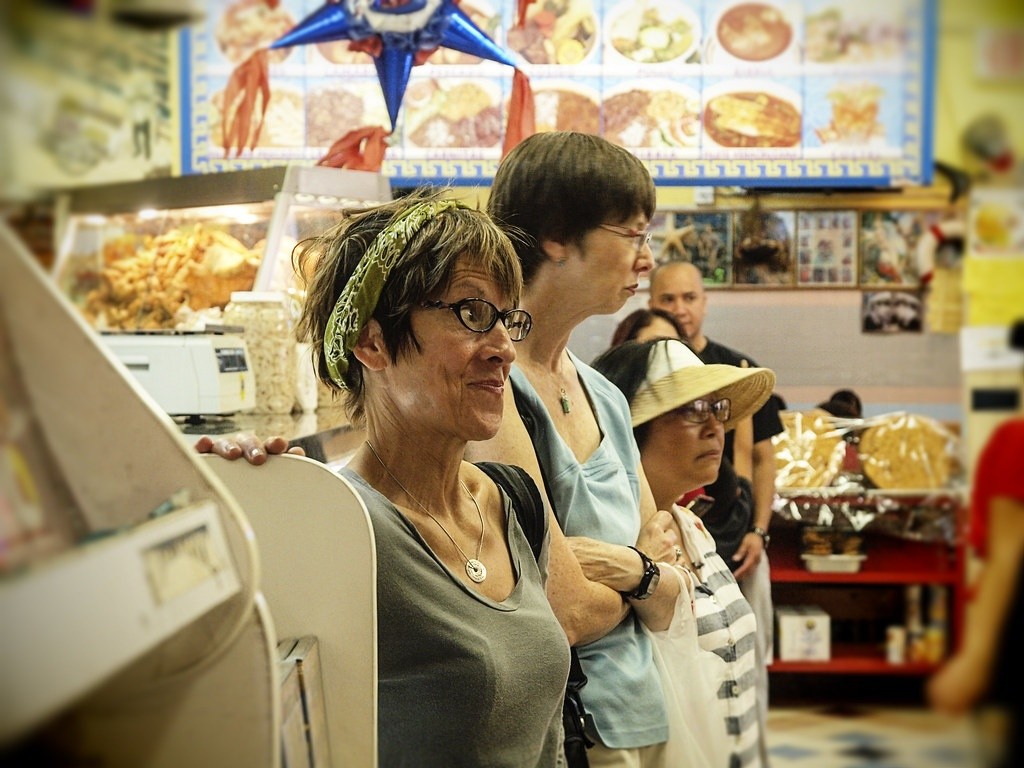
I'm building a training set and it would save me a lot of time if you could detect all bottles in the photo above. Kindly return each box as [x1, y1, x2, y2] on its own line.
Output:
[224, 291, 296, 415]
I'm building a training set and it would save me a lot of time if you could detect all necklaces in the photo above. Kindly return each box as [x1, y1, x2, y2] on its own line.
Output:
[512, 336, 570, 414]
[363, 438, 488, 583]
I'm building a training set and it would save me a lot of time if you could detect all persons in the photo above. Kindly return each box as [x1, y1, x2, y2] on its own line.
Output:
[319, 124, 391, 171]
[922, 321, 1024, 768]
[475, 132, 693, 768]
[587, 257, 785, 768]
[191, 195, 570, 767]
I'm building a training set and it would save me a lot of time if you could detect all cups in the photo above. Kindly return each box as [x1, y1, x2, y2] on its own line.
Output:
[906, 585, 946, 664]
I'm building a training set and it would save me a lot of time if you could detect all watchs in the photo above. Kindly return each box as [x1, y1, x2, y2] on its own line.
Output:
[621, 543, 660, 600]
[750, 526, 770, 548]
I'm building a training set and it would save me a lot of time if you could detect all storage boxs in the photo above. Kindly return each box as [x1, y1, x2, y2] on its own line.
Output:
[775, 602, 831, 661]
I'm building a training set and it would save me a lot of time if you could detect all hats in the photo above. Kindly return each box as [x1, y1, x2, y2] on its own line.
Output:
[627, 340, 776, 433]
[818, 391, 861, 418]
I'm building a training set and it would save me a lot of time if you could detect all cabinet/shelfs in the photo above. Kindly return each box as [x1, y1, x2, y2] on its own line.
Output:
[763, 421, 967, 675]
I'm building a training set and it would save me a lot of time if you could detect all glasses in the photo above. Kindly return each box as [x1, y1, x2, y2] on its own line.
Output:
[593, 222, 652, 247]
[674, 398, 732, 423]
[414, 297, 533, 342]
